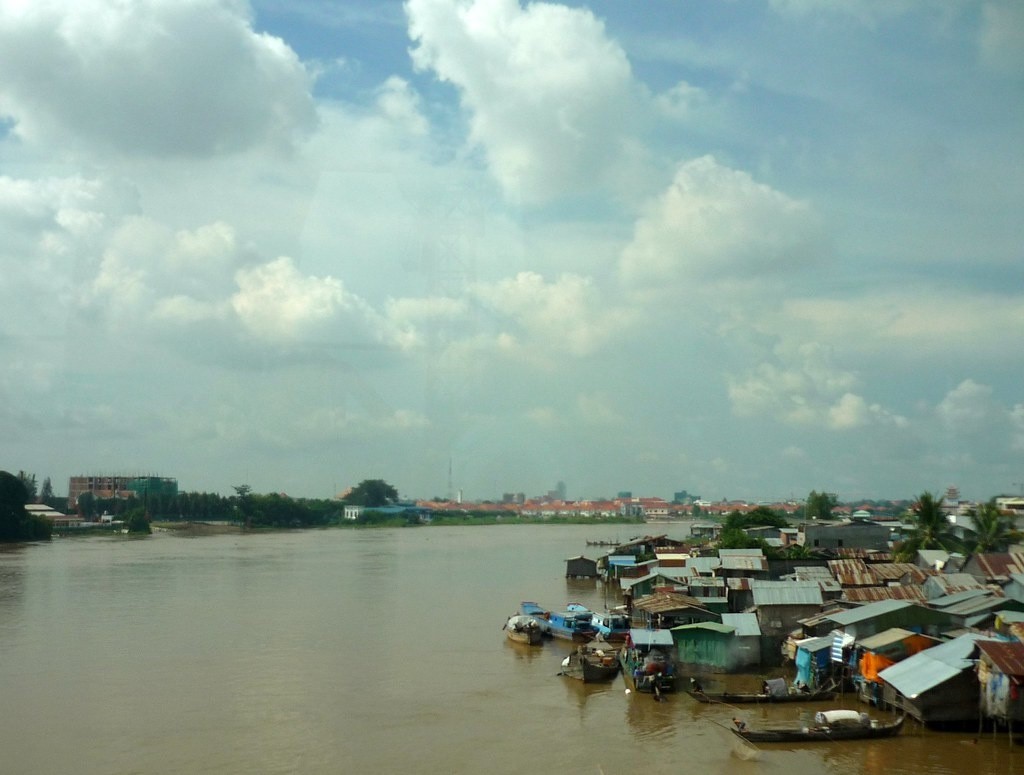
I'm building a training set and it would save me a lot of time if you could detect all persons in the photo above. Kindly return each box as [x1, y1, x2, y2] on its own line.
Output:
[545, 610, 551, 620]
[733, 717, 748, 731]
[690, 678, 704, 694]
[623, 631, 631, 663]
[797, 680, 811, 695]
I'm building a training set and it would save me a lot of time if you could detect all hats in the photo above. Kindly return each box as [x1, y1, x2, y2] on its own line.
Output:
[691, 678, 695, 683]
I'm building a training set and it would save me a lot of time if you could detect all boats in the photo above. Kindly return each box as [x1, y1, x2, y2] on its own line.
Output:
[566, 602, 633, 641]
[730, 710, 908, 742]
[618, 635, 676, 700]
[518, 603, 600, 644]
[585, 539, 621, 546]
[560, 632, 620, 680]
[502, 612, 543, 647]
[685, 677, 838, 703]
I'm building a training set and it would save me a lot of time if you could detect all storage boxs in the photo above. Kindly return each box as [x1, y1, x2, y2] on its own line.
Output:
[603, 656, 612, 666]
